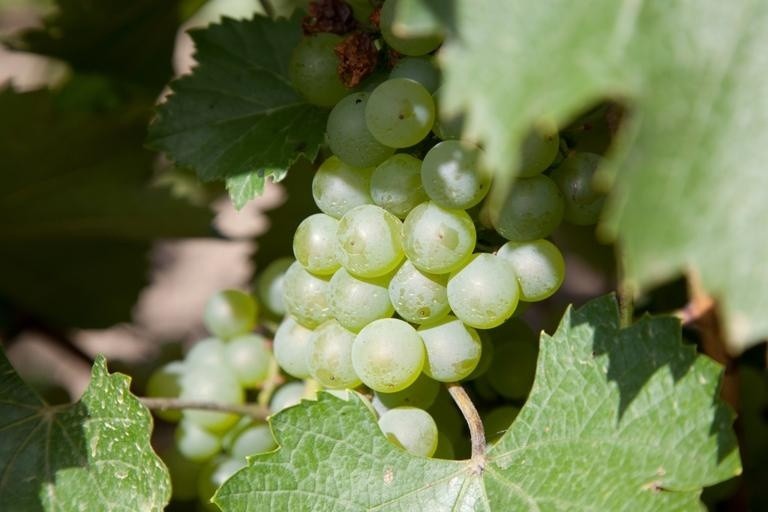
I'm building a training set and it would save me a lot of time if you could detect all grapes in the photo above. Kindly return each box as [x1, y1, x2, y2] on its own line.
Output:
[145, 0, 609, 511]
